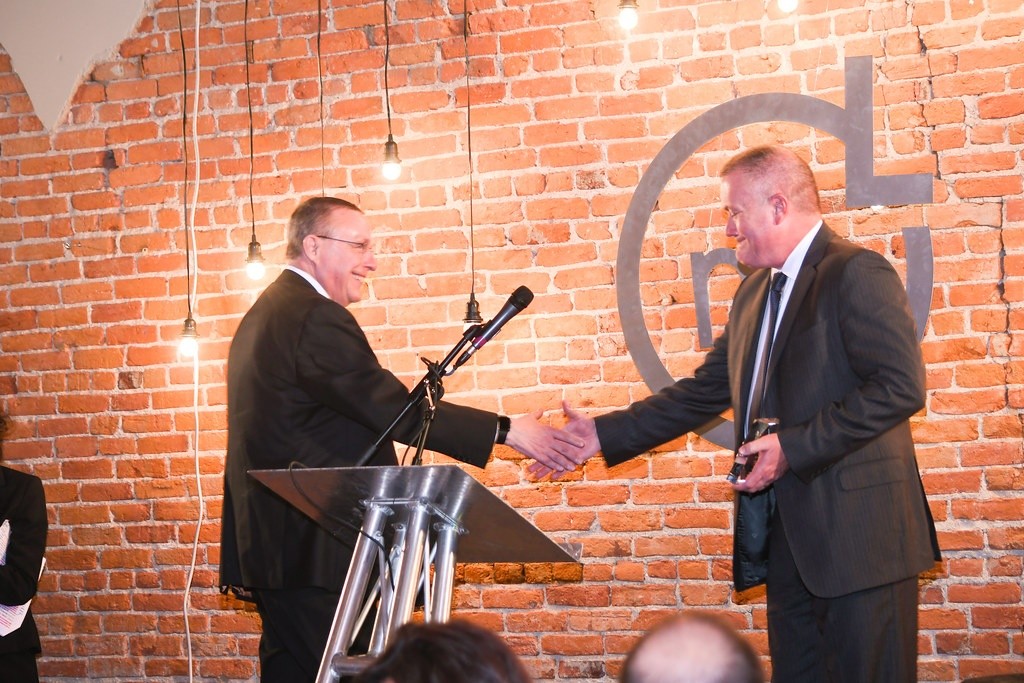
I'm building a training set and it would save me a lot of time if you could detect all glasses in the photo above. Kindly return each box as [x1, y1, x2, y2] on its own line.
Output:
[317, 235, 376, 253]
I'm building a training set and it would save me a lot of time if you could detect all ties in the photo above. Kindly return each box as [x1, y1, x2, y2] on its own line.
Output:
[747, 272, 787, 443]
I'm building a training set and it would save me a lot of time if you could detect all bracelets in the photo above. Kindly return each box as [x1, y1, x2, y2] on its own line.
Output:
[496, 415, 511, 443]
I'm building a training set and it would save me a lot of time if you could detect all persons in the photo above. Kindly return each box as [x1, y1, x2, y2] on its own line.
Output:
[524, 145, 946, 682]
[616, 608, 768, 683]
[1, 409, 50, 683]
[216, 199, 585, 683]
[342, 614, 528, 683]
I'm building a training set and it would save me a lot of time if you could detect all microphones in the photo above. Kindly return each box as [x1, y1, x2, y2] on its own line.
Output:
[455, 286, 534, 368]
